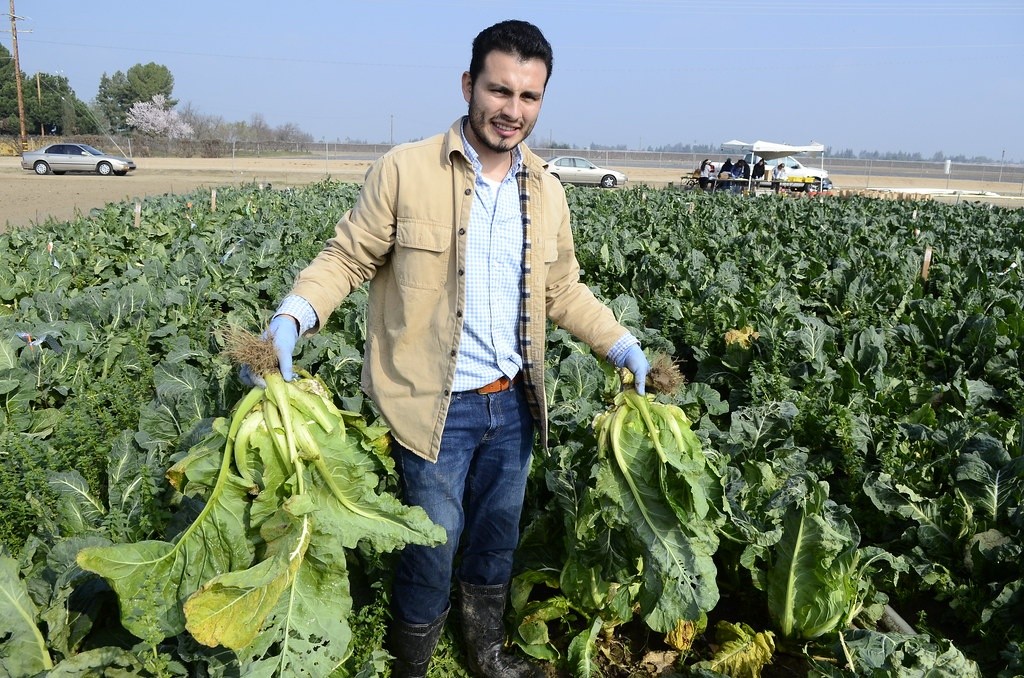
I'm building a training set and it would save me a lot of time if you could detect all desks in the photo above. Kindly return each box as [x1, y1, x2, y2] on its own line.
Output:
[711, 178, 814, 194]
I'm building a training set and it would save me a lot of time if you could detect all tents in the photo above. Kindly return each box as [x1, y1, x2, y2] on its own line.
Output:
[712, 140, 824, 193]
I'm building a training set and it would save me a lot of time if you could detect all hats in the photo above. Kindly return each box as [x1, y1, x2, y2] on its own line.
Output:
[779, 163, 785, 168]
[705, 159, 711, 163]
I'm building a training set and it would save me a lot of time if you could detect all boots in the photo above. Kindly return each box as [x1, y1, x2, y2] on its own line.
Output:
[393, 599, 451, 678]
[456, 582, 533, 678]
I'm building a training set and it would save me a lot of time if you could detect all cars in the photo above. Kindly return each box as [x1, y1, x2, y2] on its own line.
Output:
[21, 143, 137, 176]
[546, 156, 628, 188]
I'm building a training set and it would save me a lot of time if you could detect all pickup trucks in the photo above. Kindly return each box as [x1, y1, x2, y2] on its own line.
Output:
[699, 152, 833, 192]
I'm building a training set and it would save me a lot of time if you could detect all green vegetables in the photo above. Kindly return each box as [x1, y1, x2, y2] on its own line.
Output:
[571, 365, 718, 634]
[77, 317, 449, 678]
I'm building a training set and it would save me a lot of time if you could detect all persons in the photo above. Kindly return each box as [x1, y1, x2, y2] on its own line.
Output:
[238, 18, 653, 678]
[700, 158, 787, 193]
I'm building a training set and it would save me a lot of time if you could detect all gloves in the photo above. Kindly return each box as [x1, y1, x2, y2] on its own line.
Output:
[614, 343, 650, 396]
[241, 318, 299, 389]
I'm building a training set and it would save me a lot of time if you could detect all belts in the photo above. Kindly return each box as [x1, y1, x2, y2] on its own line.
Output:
[477, 377, 510, 394]
